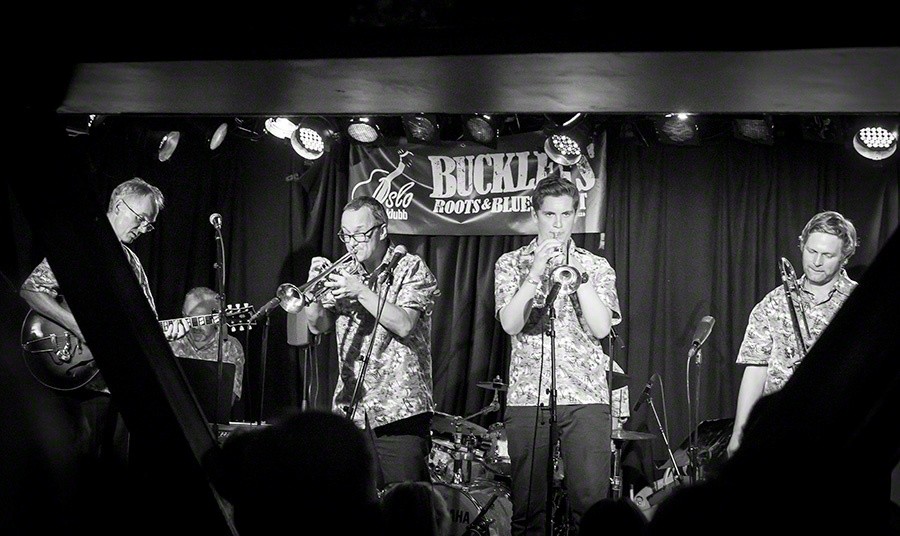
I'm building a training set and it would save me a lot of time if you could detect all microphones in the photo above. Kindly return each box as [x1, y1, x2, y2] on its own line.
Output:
[210, 213, 222, 230]
[634, 374, 657, 411]
[547, 282, 562, 307]
[250, 296, 282, 322]
[379, 244, 407, 284]
[688, 316, 716, 358]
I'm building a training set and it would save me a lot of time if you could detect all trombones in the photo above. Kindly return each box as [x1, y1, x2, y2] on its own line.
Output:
[779, 256, 812, 373]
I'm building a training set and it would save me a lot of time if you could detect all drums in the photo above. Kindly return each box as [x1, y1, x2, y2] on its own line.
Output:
[432, 438, 485, 488]
[432, 477, 512, 536]
[489, 422, 561, 474]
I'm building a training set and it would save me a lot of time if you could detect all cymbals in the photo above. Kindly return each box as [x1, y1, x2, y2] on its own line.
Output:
[430, 410, 488, 435]
[606, 371, 630, 377]
[475, 381, 508, 390]
[611, 429, 658, 440]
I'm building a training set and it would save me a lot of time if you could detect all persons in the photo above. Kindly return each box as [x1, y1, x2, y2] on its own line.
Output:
[727, 210, 858, 457]
[169, 287, 246, 406]
[305, 196, 437, 487]
[495, 176, 622, 535]
[21, 177, 163, 479]
[218, 412, 739, 536]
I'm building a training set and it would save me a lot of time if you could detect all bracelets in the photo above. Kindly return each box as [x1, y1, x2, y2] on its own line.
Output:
[525, 276, 538, 286]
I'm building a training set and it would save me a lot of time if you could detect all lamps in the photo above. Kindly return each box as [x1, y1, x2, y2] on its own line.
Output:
[209, 118, 259, 150]
[543, 116, 590, 166]
[159, 131, 197, 162]
[853, 112, 900, 161]
[402, 114, 440, 145]
[465, 114, 504, 149]
[732, 114, 773, 147]
[347, 117, 383, 143]
[288, 117, 336, 161]
[655, 113, 702, 144]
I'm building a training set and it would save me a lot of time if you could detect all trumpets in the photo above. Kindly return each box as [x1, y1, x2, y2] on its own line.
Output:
[277, 249, 358, 314]
[546, 234, 582, 294]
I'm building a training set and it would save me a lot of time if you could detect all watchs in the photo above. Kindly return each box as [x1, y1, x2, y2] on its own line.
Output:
[580, 271, 589, 284]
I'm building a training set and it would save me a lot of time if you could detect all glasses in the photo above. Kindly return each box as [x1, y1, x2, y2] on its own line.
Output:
[337, 222, 385, 243]
[116, 199, 155, 233]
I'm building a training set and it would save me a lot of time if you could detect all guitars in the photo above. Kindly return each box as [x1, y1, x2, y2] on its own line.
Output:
[21, 295, 257, 391]
